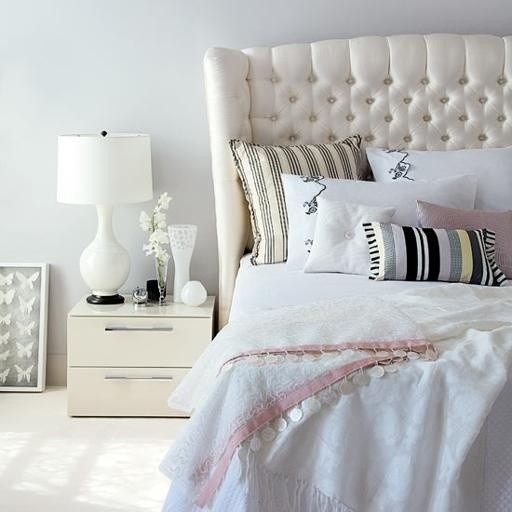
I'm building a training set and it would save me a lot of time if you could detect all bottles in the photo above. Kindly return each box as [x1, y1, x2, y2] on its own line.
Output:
[181, 280, 208, 307]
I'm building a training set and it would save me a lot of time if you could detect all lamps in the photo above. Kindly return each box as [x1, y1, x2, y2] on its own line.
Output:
[54, 131, 155, 304]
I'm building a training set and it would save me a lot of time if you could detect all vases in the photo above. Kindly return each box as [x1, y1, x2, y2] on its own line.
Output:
[154, 257, 167, 306]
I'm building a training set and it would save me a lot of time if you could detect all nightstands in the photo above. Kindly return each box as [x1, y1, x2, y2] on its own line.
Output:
[65, 294, 217, 418]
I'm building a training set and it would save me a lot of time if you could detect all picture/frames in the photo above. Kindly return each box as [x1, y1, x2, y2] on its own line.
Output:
[0, 262, 49, 394]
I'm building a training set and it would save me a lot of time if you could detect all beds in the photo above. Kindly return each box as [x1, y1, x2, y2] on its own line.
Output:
[201, 32, 512, 511]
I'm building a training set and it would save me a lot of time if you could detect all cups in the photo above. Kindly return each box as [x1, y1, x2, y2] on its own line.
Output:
[147, 279, 166, 302]
[167, 224, 198, 303]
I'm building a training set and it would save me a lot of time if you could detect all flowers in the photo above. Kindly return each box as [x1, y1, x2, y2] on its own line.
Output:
[140, 191, 171, 288]
